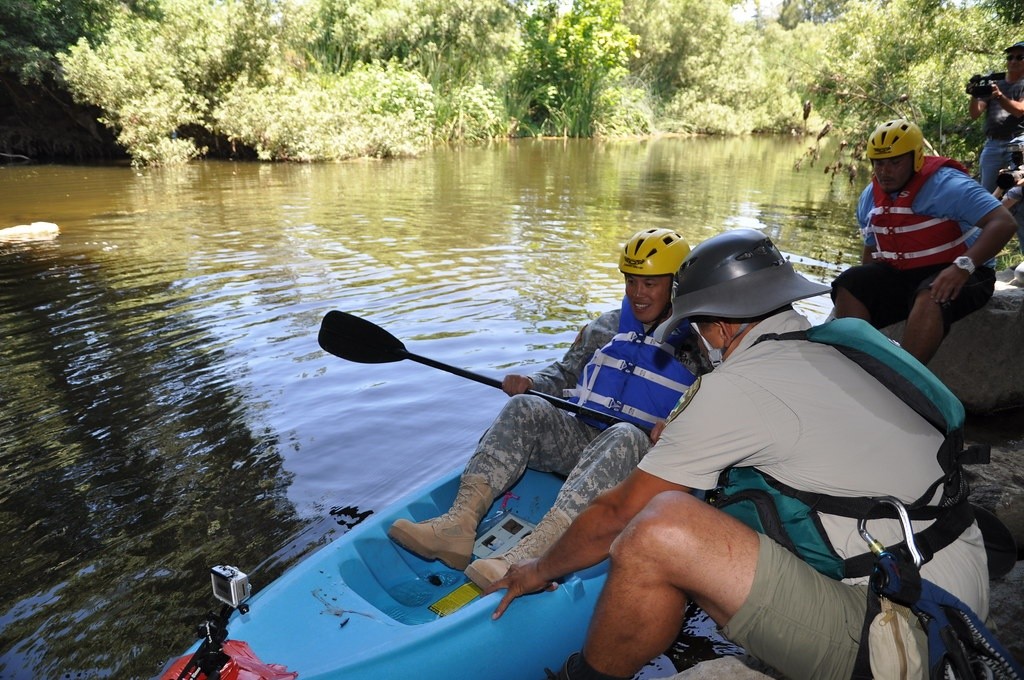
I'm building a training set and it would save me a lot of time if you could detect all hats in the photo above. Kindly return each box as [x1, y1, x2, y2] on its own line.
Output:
[1005, 41, 1024, 51]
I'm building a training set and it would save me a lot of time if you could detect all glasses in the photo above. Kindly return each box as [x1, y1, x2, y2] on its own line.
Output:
[1007, 55, 1024, 61]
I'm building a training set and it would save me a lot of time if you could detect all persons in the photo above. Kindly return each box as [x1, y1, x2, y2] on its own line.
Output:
[992, 135, 1024, 282]
[969, 40, 1024, 195]
[480, 229, 990, 680]
[386, 228, 715, 591]
[830, 119, 1019, 366]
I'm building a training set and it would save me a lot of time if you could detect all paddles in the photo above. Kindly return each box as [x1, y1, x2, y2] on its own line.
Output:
[317, 309, 651, 437]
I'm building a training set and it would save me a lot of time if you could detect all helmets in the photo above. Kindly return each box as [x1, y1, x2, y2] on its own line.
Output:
[653, 228, 832, 344]
[867, 119, 924, 172]
[618, 228, 692, 276]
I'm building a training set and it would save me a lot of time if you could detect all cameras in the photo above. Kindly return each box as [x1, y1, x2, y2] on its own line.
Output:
[997, 152, 1023, 191]
[210, 567, 250, 608]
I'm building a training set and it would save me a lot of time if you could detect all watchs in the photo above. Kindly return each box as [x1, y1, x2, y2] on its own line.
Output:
[954, 256, 975, 274]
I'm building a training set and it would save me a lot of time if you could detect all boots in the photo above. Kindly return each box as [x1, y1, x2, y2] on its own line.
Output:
[464, 506, 573, 594]
[389, 474, 501, 571]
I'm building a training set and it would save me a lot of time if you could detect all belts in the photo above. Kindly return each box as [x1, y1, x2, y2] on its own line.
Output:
[988, 133, 1014, 140]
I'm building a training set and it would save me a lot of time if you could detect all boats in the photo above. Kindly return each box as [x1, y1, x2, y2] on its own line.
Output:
[149, 451, 611, 680]
